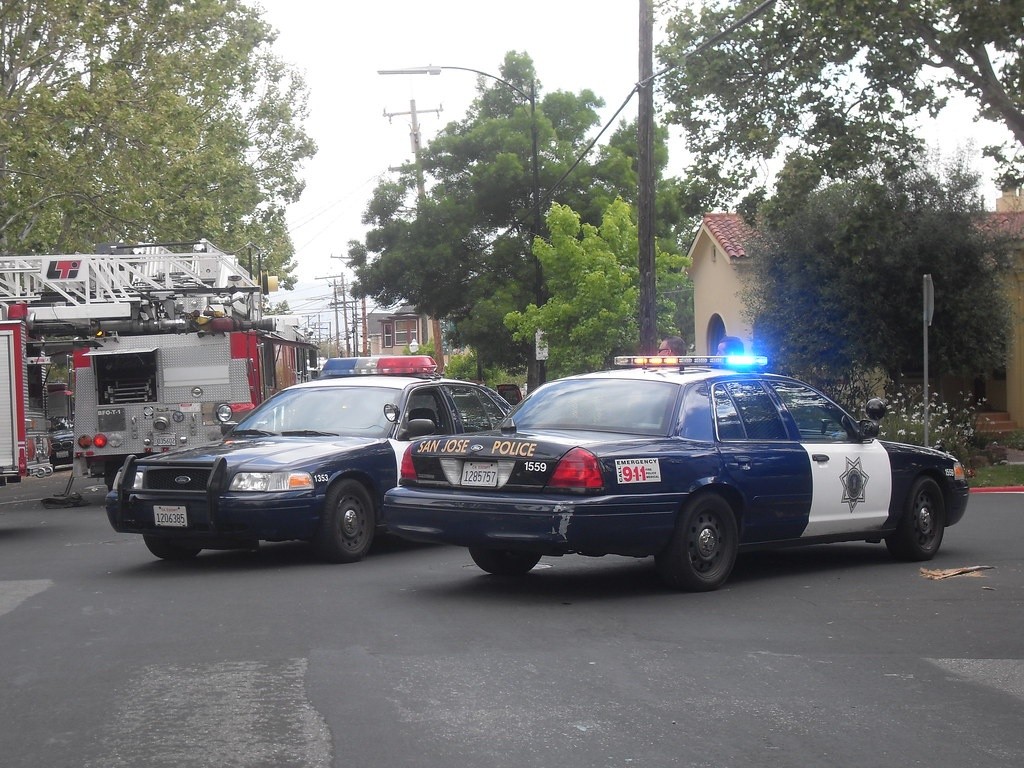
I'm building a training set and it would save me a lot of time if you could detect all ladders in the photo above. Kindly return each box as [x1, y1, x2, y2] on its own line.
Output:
[0, 236, 252, 304]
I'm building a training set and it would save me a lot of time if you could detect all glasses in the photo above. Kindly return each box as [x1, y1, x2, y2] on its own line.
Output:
[658, 348, 669, 353]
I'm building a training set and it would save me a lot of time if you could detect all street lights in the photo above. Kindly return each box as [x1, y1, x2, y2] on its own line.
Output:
[376, 66, 542, 402]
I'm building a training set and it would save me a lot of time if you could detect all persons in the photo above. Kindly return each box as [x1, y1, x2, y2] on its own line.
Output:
[656, 336, 687, 356]
[716, 335, 744, 357]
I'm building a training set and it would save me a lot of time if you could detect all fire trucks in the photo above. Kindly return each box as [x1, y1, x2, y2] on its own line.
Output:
[0, 253, 321, 511]
[0, 300, 29, 486]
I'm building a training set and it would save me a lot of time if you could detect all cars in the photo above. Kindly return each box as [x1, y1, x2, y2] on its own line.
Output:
[104, 352, 531, 564]
[383, 354, 974, 593]
[48, 428, 75, 470]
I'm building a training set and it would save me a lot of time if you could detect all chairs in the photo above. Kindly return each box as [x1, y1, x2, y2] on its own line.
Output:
[408, 408, 438, 427]
